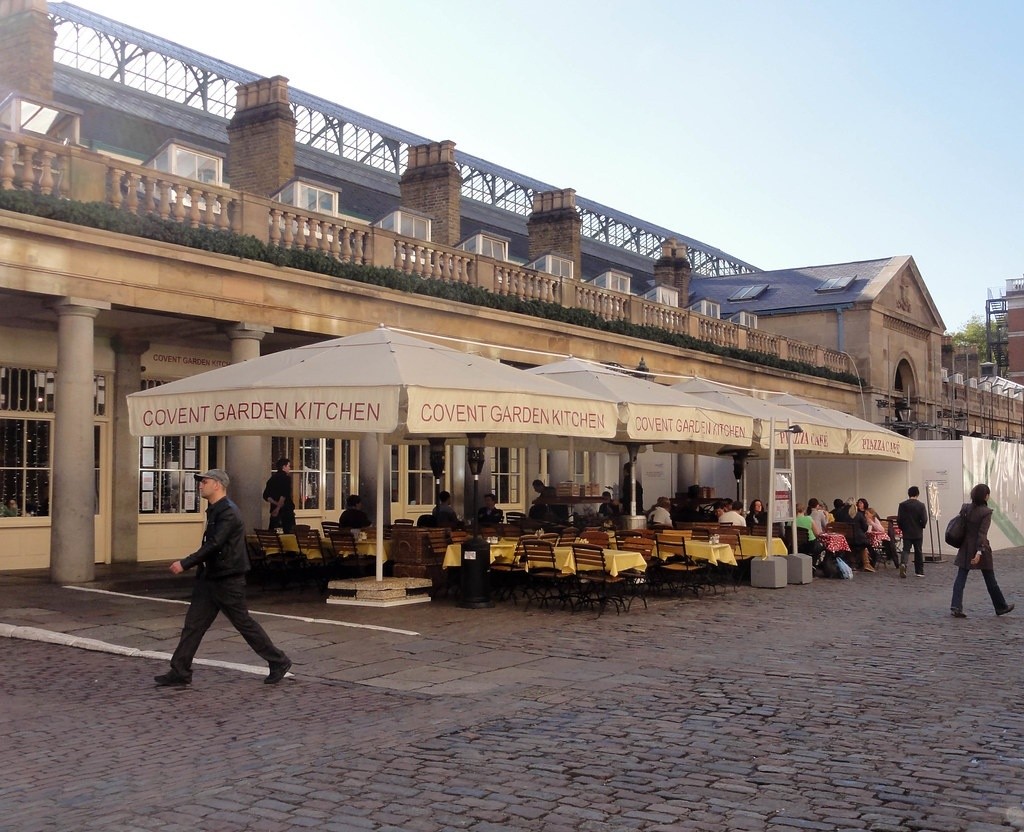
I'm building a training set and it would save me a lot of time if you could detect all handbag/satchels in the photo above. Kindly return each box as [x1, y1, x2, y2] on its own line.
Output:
[834, 556, 853, 580]
[945, 502, 970, 549]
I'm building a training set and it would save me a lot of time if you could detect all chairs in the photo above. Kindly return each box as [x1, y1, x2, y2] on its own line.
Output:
[252, 514, 901, 618]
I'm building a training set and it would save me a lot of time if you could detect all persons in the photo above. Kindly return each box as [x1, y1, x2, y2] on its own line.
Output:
[339, 495, 372, 528]
[263, 459, 297, 535]
[153, 468, 292, 685]
[786, 497, 886, 572]
[949, 484, 1015, 618]
[531, 479, 568, 521]
[432, 491, 457, 521]
[646, 496, 673, 527]
[613, 463, 644, 515]
[896, 486, 928, 578]
[477, 493, 503, 524]
[709, 498, 768, 527]
[598, 492, 619, 516]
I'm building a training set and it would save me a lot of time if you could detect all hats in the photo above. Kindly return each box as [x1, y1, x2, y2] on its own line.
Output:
[193, 468, 230, 487]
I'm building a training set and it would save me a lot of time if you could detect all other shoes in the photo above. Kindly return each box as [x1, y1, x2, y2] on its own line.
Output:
[263, 659, 293, 684]
[996, 604, 1016, 616]
[862, 565, 876, 573]
[915, 571, 926, 577]
[949, 609, 966, 618]
[154, 674, 182, 686]
[898, 563, 908, 578]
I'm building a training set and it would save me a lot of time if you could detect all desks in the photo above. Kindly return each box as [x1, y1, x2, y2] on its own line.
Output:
[530, 545, 644, 615]
[635, 539, 734, 594]
[326, 537, 393, 576]
[812, 532, 849, 559]
[251, 533, 333, 586]
[441, 538, 527, 599]
[715, 532, 788, 587]
[861, 531, 890, 545]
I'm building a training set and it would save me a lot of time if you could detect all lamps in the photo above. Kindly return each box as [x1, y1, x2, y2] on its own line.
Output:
[876, 397, 913, 409]
[783, 425, 804, 434]
[938, 405, 971, 420]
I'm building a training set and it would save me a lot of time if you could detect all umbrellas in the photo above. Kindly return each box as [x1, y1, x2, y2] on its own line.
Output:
[664, 376, 913, 517]
[125, 323, 618, 578]
[524, 355, 754, 518]
